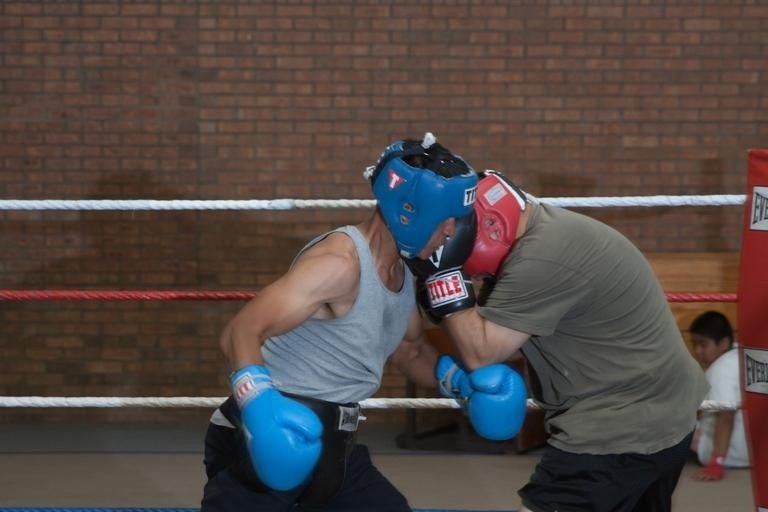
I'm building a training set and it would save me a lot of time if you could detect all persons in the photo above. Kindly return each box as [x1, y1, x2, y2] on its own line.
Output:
[400, 167, 714, 510]
[200, 132, 527, 509]
[684, 309, 750, 484]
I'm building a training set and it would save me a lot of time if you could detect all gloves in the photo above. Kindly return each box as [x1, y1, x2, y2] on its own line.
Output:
[407, 210, 475, 325]
[229, 363, 324, 493]
[435, 355, 528, 441]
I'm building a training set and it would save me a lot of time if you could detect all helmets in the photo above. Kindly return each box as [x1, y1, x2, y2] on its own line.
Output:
[369, 140, 478, 261]
[463, 169, 525, 276]
